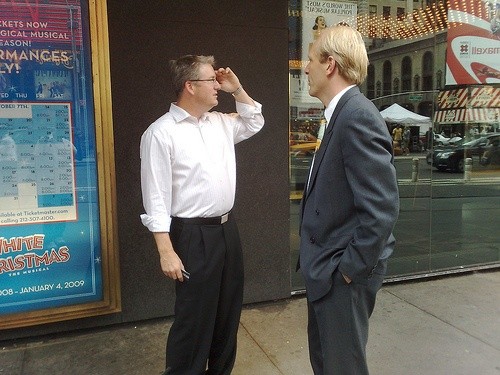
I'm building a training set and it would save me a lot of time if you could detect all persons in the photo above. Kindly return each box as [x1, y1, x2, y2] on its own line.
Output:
[140, 55, 266, 375]
[426, 126, 434, 149]
[441, 127, 494, 137]
[294, 24, 398, 375]
[392, 124, 411, 156]
[313, 16, 327, 40]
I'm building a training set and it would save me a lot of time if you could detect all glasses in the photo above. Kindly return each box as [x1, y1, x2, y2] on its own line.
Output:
[188, 77, 216, 83]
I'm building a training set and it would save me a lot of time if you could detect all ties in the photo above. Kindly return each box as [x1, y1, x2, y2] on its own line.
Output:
[315, 115, 327, 154]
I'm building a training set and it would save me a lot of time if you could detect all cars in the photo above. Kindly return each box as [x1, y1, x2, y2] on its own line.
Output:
[289, 131, 319, 157]
[418, 132, 500, 173]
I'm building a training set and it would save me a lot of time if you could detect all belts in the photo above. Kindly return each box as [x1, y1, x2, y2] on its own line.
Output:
[172, 208, 232, 226]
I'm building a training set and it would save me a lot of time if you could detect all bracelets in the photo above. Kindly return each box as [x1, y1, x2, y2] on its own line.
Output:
[232, 85, 243, 97]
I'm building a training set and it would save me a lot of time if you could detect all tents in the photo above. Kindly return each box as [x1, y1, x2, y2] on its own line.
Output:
[379, 102, 434, 151]
